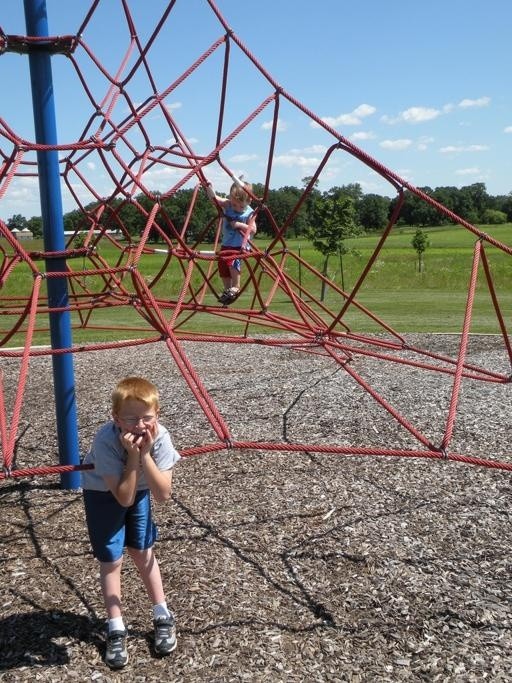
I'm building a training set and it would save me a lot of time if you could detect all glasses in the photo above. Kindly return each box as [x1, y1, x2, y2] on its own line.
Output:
[116, 416, 157, 425]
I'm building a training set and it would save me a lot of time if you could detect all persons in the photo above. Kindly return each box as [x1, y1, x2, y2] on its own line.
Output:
[206, 174, 257, 303]
[82, 377, 182, 669]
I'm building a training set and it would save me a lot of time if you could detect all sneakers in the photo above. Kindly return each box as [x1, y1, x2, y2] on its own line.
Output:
[105, 628, 129, 668]
[152, 611, 177, 655]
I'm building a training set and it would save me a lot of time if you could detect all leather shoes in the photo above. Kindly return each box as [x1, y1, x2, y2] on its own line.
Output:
[218, 287, 240, 304]
